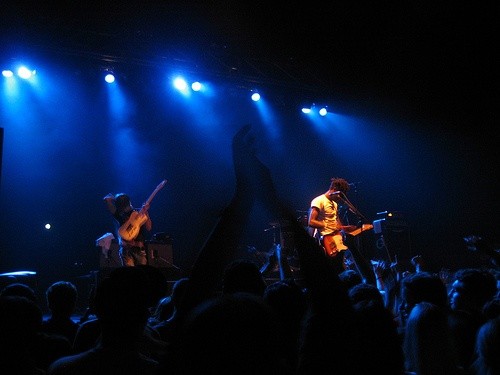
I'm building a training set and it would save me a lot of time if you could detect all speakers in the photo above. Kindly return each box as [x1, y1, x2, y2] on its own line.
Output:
[145, 244, 173, 269]
[356, 226, 412, 269]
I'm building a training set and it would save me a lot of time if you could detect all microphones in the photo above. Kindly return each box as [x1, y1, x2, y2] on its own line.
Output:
[329, 190, 341, 196]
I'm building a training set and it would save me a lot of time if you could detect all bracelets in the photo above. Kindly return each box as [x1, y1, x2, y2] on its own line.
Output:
[324, 223, 327, 228]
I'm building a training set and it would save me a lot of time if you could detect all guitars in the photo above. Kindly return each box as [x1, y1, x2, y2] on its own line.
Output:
[321, 222, 375, 257]
[118, 181, 166, 243]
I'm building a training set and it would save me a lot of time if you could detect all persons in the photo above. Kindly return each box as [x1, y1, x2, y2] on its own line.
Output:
[103, 192, 151, 265]
[308, 177, 370, 272]
[0, 124, 500, 375]
